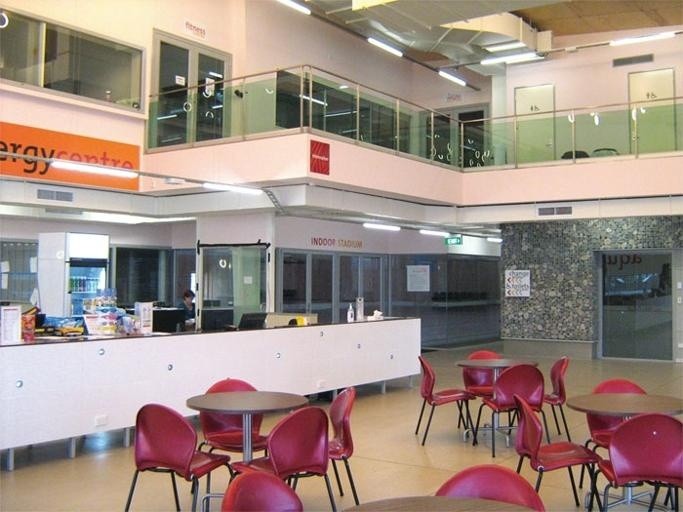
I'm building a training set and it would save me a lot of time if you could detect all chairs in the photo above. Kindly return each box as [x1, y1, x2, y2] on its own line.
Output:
[561, 147, 618, 161]
[414, 356, 479, 446]
[580, 379, 647, 488]
[509, 356, 572, 443]
[472, 364, 551, 458]
[435, 464, 545, 512]
[587, 413, 683, 511]
[512, 394, 605, 512]
[461, 348, 507, 437]
[124, 379, 359, 512]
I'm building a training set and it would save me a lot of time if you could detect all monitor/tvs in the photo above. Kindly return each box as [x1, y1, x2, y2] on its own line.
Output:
[201, 308, 234, 331]
[238, 312, 267, 330]
[152, 309, 185, 333]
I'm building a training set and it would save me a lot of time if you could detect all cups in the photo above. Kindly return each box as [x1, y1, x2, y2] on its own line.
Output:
[21, 315, 35, 343]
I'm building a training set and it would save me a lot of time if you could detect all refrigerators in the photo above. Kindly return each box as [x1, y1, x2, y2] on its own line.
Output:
[34, 232, 110, 319]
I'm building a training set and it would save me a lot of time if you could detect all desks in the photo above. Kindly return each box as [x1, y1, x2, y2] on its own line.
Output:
[341, 495, 536, 512]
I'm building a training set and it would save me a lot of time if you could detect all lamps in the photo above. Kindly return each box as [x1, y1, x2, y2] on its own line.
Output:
[485, 236, 502, 243]
[50, 159, 137, 180]
[202, 182, 262, 197]
[419, 229, 450, 238]
[275, 0, 675, 87]
[361, 222, 400, 232]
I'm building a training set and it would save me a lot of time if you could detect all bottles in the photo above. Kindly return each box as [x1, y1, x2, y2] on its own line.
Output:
[347, 303, 355, 323]
[69, 295, 83, 315]
[68, 275, 101, 293]
[105, 91, 112, 101]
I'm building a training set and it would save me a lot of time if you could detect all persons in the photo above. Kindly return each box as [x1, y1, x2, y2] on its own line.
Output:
[175, 289, 195, 320]
